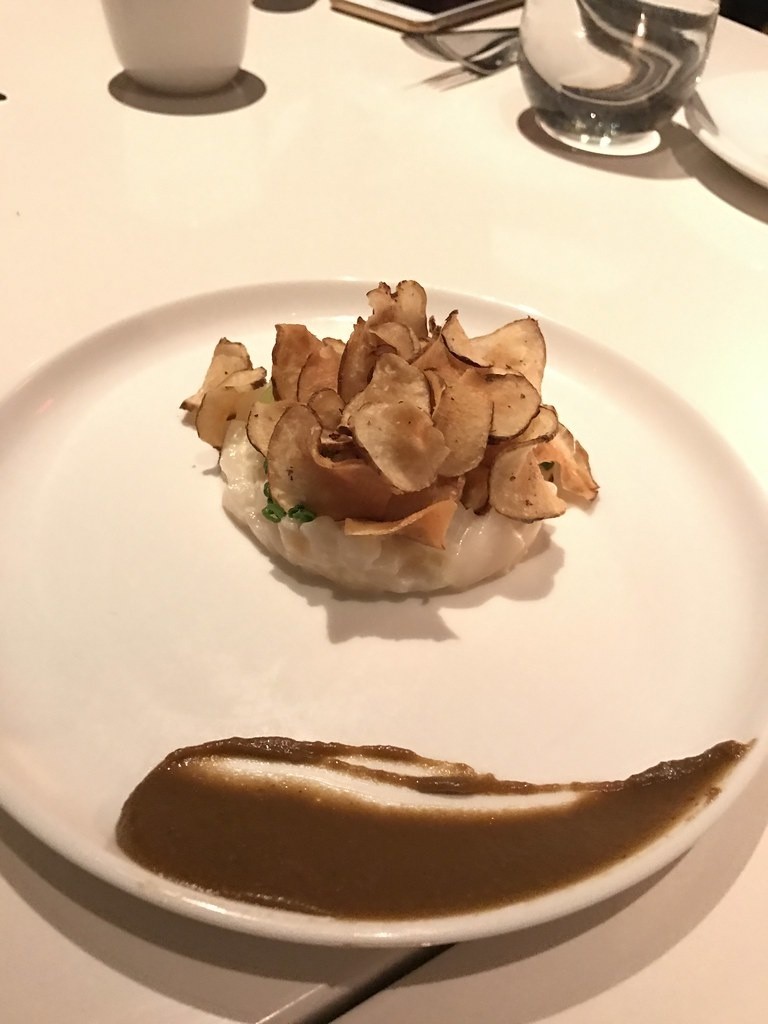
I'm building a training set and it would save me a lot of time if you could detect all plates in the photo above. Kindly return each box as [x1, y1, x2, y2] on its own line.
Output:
[683, 72, 768, 188]
[1, 278, 768, 950]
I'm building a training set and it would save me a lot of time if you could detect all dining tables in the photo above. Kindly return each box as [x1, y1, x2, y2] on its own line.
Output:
[0, 0, 768, 1024]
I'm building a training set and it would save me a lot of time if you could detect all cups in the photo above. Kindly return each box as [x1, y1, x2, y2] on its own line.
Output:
[520, 1, 720, 157]
[107, 0, 250, 98]
